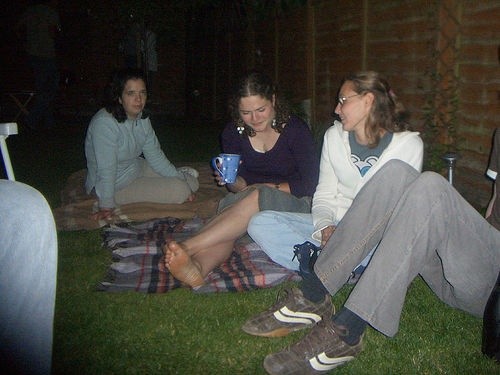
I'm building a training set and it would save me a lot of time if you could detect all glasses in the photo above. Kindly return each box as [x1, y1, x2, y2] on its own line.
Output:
[338, 94, 359, 104]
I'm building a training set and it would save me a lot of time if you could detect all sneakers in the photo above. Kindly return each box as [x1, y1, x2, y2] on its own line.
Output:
[241, 283, 336, 340]
[263, 315, 367, 375]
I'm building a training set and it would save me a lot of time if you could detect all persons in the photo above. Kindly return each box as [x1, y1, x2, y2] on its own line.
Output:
[0, 179, 58, 375]
[84, 70, 199, 221]
[242, 128, 500, 375]
[247, 71, 424, 282]
[157, 69, 319, 292]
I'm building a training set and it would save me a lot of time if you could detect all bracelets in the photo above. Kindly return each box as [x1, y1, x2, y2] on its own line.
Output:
[275, 183, 280, 189]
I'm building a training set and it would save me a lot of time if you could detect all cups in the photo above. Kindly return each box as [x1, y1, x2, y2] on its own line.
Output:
[212, 154, 241, 184]
